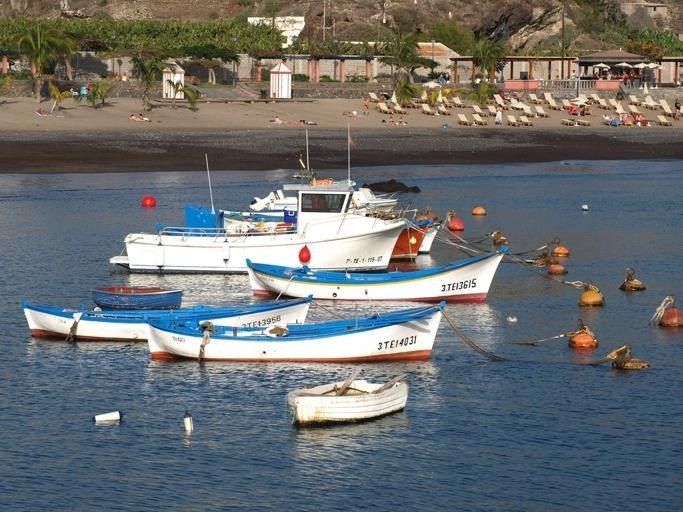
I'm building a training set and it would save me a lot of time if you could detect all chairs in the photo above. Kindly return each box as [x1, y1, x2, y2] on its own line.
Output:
[369, 91, 683, 127]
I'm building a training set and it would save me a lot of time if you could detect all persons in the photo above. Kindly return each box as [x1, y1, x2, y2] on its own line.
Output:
[603, 114, 610, 127]
[128, 113, 145, 122]
[138, 113, 151, 122]
[569, 68, 577, 88]
[259, 87, 266, 99]
[516, 90, 524, 102]
[362, 96, 369, 115]
[113, 71, 121, 80]
[506, 90, 512, 102]
[69, 88, 82, 108]
[122, 73, 129, 81]
[0, 54, 8, 76]
[674, 99, 680, 120]
[380, 115, 407, 127]
[84, 78, 92, 101]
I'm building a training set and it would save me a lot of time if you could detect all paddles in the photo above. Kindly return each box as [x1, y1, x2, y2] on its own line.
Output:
[369, 374, 407, 393]
[336, 369, 361, 396]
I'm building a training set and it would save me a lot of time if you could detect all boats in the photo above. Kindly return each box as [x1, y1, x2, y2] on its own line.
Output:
[17, 293, 312, 346]
[144, 300, 447, 364]
[90, 285, 183, 312]
[109, 119, 451, 278]
[285, 378, 410, 427]
[243, 244, 511, 303]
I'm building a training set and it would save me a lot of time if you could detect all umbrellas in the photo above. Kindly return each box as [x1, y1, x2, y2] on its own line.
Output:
[592, 62, 665, 86]
